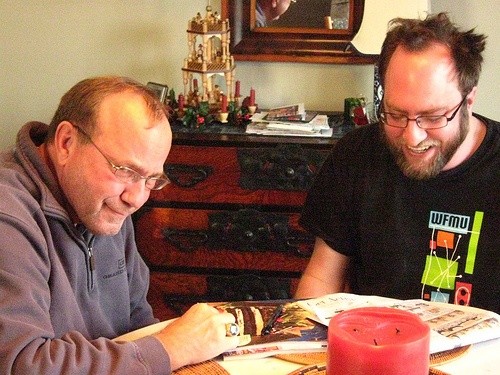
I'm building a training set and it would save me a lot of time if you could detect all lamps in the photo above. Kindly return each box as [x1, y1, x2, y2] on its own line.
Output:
[351, 0, 430, 54]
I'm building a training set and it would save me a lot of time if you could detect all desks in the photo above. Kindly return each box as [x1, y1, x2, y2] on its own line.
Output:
[111, 299, 500, 375]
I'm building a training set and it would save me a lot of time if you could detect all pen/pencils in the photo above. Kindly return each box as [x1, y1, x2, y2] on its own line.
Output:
[261, 304, 282, 336]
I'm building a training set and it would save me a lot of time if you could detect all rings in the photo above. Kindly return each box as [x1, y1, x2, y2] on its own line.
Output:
[226, 323, 240, 337]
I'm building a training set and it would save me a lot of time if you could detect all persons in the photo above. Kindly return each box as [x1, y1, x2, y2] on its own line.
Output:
[292, 17, 500, 314]
[0, 77, 251, 375]
[254, 1, 296, 26]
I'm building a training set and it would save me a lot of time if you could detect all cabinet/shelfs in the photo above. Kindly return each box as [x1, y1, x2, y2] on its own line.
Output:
[131, 109, 351, 321]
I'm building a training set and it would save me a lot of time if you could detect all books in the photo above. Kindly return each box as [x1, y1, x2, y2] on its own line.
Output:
[197, 300, 328, 362]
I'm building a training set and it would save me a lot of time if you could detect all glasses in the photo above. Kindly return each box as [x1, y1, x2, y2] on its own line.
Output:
[377, 94, 467, 129]
[72, 124, 171, 191]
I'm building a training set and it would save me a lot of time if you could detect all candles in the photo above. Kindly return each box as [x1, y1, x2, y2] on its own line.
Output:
[221, 95, 227, 112]
[251, 90, 255, 106]
[235, 81, 240, 97]
[193, 79, 198, 93]
[328, 307, 430, 375]
[178, 93, 184, 111]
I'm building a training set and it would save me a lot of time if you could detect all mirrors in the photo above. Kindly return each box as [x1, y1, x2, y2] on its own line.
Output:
[220, 0, 377, 65]
